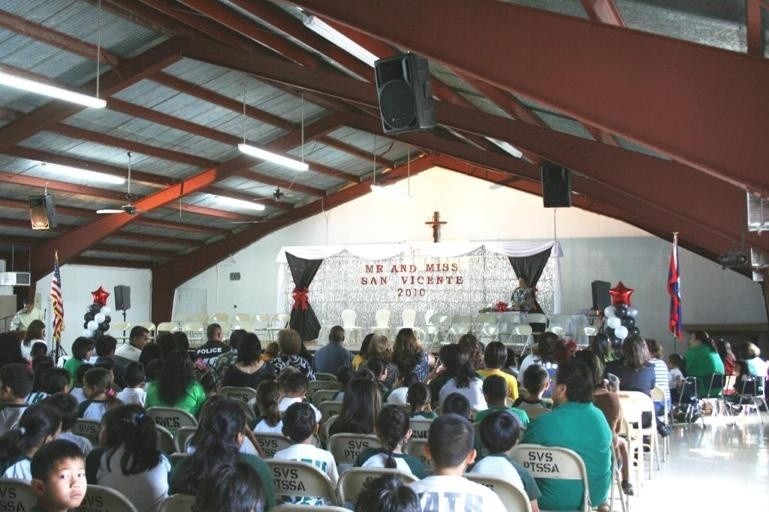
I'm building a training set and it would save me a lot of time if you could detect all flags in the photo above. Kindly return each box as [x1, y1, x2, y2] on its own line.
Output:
[666, 238, 684, 340]
[49, 255, 66, 342]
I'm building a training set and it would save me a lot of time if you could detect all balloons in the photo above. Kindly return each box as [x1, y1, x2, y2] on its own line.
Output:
[607, 280, 634, 305]
[90, 286, 110, 305]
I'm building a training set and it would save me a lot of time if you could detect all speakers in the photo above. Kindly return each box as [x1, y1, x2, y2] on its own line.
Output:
[591, 280, 611, 310]
[114, 285, 130, 310]
[29, 193, 58, 230]
[374, 53, 437, 135]
[541, 165, 571, 207]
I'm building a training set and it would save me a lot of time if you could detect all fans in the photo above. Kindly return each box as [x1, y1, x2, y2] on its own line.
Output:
[253, 185, 302, 201]
[96, 152, 161, 218]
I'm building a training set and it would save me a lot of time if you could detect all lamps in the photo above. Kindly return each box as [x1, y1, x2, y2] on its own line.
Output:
[370, 109, 414, 200]
[237, 74, 309, 172]
[302, 13, 380, 69]
[0, 0, 107, 109]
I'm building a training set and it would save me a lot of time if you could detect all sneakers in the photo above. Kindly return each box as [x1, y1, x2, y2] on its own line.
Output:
[659, 424, 669, 436]
[622, 480, 633, 495]
[692, 410, 705, 422]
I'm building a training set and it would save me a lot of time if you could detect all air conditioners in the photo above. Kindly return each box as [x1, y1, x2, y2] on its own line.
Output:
[0, 272, 31, 287]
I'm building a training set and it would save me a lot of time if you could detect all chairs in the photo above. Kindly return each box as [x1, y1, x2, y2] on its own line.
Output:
[153, 425, 179, 455]
[1, 476, 36, 511]
[459, 473, 536, 512]
[328, 433, 381, 467]
[265, 459, 334, 507]
[2, 308, 769, 445]
[507, 443, 591, 511]
[613, 388, 663, 479]
[172, 425, 198, 454]
[336, 467, 418, 505]
[159, 495, 203, 511]
[251, 431, 292, 459]
[604, 416, 636, 512]
[408, 435, 446, 476]
[34, 485, 137, 512]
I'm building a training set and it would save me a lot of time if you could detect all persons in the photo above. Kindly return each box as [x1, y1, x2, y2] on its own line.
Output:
[510, 276, 535, 310]
[1, 300, 768, 511]
